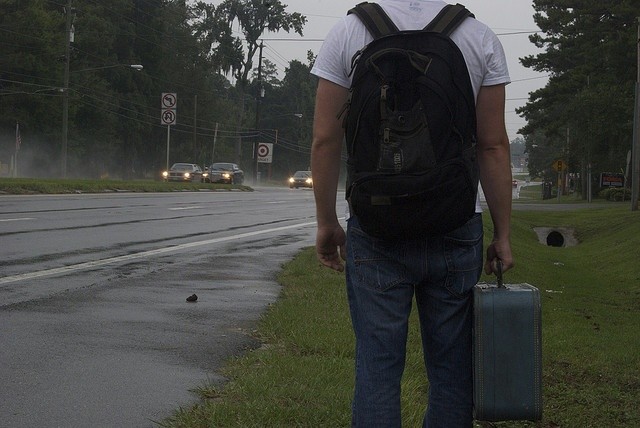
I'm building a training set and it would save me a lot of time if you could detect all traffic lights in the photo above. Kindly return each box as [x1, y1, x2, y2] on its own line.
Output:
[557, 161, 561, 171]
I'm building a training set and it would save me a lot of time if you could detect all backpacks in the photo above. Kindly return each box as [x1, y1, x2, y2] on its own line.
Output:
[335, 1, 480, 240]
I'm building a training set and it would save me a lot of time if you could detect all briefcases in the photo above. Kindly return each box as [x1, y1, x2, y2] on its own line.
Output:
[474, 259, 543, 427]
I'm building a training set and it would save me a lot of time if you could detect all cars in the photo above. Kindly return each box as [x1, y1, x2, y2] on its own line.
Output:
[163, 163, 202, 182]
[289, 171, 313, 188]
[202, 163, 243, 184]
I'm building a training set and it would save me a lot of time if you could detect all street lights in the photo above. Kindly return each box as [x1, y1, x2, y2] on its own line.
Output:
[252, 113, 302, 186]
[62, 64, 143, 177]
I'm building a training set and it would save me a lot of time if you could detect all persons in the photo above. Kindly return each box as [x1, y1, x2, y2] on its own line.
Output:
[309, 0, 514, 428]
